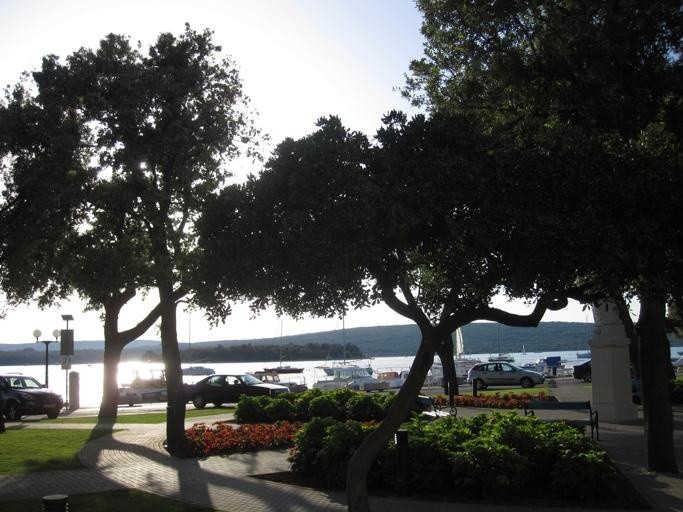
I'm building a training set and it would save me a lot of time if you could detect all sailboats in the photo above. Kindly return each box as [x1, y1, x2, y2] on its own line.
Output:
[182, 308, 215, 375]
[430, 328, 482, 379]
[265, 321, 304, 373]
[323, 316, 373, 376]
[488, 327, 514, 362]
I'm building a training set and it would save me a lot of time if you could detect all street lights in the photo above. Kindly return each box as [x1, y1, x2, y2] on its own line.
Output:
[33, 328, 61, 389]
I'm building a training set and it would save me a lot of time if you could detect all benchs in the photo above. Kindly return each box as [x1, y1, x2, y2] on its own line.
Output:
[524, 400, 599, 442]
[414, 394, 458, 421]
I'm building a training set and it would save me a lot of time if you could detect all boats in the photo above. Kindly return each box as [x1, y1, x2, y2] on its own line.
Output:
[118, 369, 188, 404]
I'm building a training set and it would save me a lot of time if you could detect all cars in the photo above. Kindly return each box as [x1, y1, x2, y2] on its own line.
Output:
[186, 374, 290, 408]
[0, 372, 66, 420]
[573, 360, 591, 382]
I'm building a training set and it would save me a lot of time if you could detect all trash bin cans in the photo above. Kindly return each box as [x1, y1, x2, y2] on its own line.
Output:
[42, 495, 69, 512]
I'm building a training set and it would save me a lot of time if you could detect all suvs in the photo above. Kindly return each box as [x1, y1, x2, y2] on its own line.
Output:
[467, 362, 545, 390]
